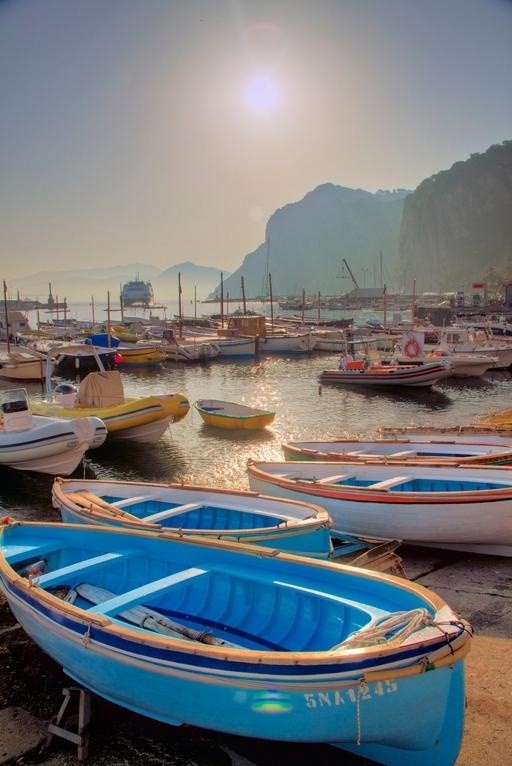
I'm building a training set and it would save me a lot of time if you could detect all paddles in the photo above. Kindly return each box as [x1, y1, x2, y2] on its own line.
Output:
[64, 488, 147, 523]
[77, 580, 247, 649]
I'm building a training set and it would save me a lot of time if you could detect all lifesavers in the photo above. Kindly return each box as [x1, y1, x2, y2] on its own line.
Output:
[403, 340, 420, 356]
